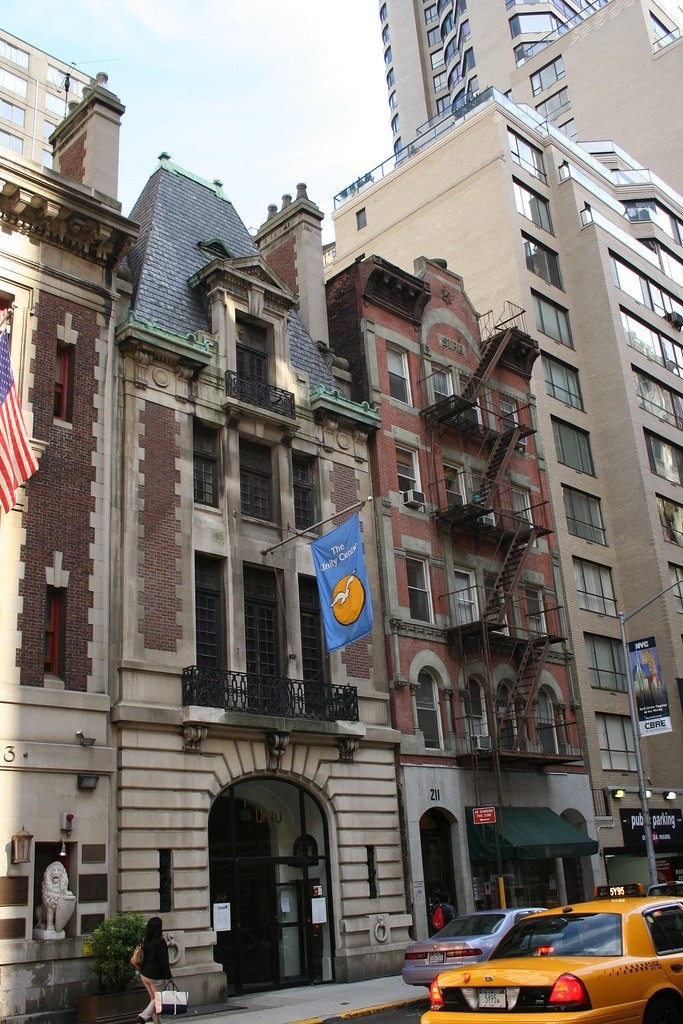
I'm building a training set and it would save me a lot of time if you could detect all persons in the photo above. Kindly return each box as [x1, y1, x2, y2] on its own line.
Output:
[138, 916, 175, 1024]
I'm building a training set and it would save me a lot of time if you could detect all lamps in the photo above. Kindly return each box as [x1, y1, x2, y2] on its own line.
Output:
[77, 775, 100, 789]
[612, 788, 627, 799]
[12, 825, 34, 862]
[639, 789, 653, 800]
[76, 729, 96, 746]
[59, 840, 67, 857]
[662, 791, 678, 800]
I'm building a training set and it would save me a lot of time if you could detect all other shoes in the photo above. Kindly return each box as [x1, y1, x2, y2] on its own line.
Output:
[136, 1016, 145, 1024]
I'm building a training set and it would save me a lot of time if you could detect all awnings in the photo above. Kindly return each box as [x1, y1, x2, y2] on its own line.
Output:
[464, 806, 599, 864]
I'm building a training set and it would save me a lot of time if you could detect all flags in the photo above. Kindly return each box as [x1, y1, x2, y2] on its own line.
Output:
[0, 324, 40, 514]
[307, 509, 374, 655]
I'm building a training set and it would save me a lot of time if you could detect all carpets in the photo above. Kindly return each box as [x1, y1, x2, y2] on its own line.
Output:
[161, 1004, 249, 1019]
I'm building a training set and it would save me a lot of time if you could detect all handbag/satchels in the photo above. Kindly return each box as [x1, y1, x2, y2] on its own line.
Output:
[130, 945, 144, 969]
[155, 982, 189, 1014]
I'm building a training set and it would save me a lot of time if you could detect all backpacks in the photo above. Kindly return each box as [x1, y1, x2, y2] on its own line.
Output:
[433, 904, 444, 930]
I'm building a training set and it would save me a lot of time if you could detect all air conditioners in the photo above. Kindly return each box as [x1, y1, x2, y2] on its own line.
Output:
[477, 516, 494, 527]
[516, 437, 527, 448]
[472, 735, 492, 752]
[402, 489, 426, 508]
[670, 312, 683, 328]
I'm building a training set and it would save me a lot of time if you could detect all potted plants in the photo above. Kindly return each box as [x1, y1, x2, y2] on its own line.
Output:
[75, 903, 152, 1024]
[471, 490, 481, 503]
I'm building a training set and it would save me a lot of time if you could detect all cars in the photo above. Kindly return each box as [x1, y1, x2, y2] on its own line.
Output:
[420, 881, 683, 1024]
[473, 874, 560, 909]
[401, 908, 567, 991]
